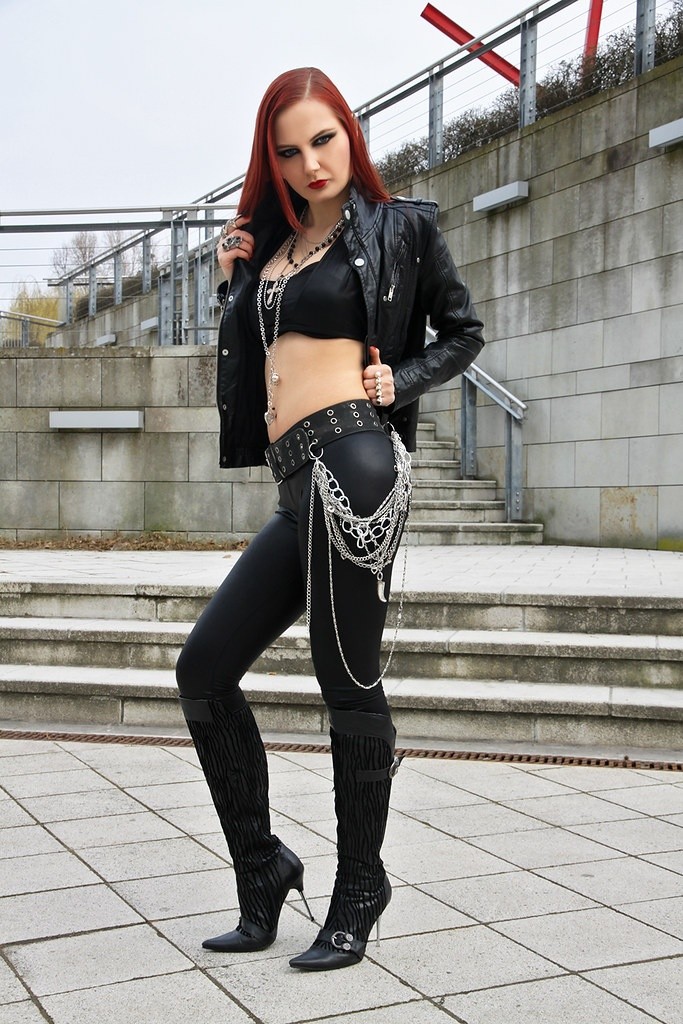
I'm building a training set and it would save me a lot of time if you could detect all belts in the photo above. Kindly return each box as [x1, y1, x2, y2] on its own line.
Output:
[265, 400, 387, 487]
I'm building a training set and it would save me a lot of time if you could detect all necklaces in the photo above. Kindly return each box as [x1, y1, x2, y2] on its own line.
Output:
[256, 207, 345, 427]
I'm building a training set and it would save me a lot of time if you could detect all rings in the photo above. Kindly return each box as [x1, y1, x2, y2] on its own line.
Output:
[219, 214, 244, 238]
[374, 370, 384, 407]
[221, 234, 242, 253]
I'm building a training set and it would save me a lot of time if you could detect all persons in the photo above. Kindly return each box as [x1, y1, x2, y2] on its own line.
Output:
[173, 64, 486, 974]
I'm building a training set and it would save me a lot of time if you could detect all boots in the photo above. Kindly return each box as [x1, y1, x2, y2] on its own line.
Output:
[288, 706, 406, 971]
[179, 694, 314, 952]
